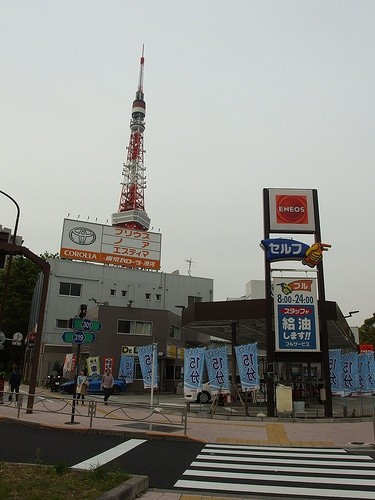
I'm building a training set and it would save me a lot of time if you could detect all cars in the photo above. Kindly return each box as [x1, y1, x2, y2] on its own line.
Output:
[60, 374, 128, 396]
[185, 373, 253, 405]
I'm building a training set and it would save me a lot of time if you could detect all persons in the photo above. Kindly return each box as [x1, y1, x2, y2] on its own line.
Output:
[313, 377, 318, 387]
[51, 376, 60, 392]
[76, 370, 88, 406]
[8, 368, 21, 401]
[102, 370, 113, 405]
[297, 376, 304, 389]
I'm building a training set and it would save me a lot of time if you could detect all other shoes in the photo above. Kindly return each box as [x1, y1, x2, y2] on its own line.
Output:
[105, 403, 108, 405]
[82, 404, 85, 406]
[76, 402, 78, 405]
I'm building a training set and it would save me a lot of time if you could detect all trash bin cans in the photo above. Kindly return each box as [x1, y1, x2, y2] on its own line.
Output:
[176, 382, 184, 394]
[276, 385, 293, 412]
[0, 374, 5, 404]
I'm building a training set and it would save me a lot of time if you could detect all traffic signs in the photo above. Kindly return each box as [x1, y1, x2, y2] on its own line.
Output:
[72, 317, 103, 332]
[62, 331, 98, 344]
